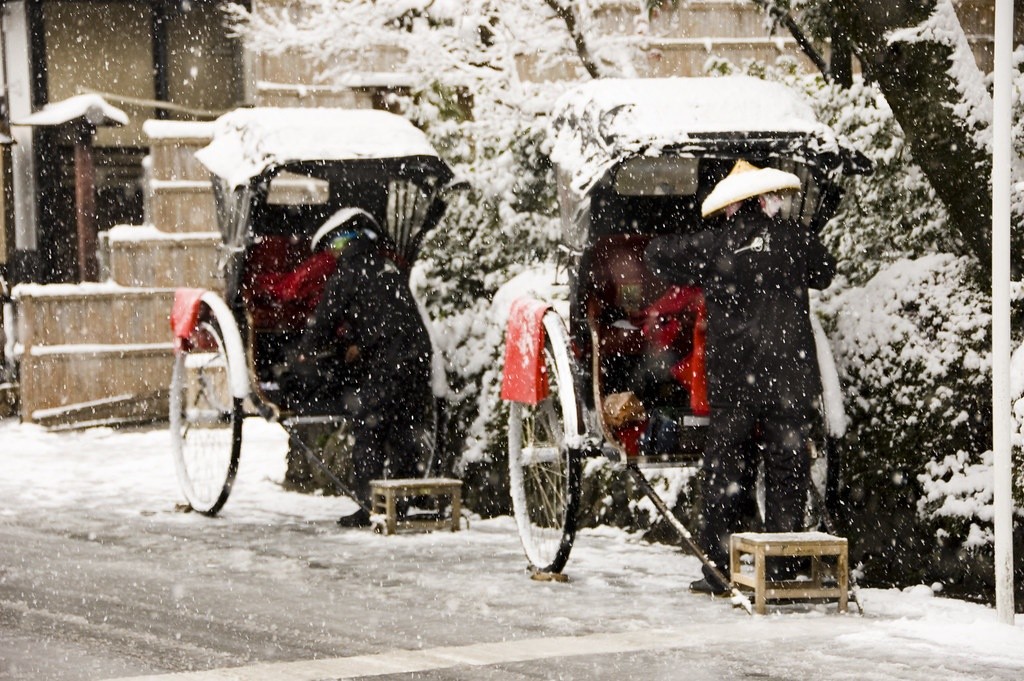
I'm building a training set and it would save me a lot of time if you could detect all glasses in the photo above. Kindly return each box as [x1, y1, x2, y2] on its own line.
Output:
[329, 233, 359, 258]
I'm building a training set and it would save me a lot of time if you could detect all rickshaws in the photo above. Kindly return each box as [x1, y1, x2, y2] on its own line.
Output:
[170, 103, 460, 533]
[504, 77, 864, 616]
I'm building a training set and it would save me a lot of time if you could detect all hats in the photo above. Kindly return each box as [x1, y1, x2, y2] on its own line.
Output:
[311, 208, 381, 252]
[701, 158, 801, 217]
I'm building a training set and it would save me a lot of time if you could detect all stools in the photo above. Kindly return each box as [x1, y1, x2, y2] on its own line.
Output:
[373, 477, 462, 536]
[730, 531, 849, 615]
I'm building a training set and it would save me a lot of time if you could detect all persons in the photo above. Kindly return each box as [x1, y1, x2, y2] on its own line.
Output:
[291, 206, 419, 528]
[644, 160, 839, 597]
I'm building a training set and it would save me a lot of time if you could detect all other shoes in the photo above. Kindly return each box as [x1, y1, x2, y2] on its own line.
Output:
[689, 578, 728, 596]
[339, 502, 373, 527]
